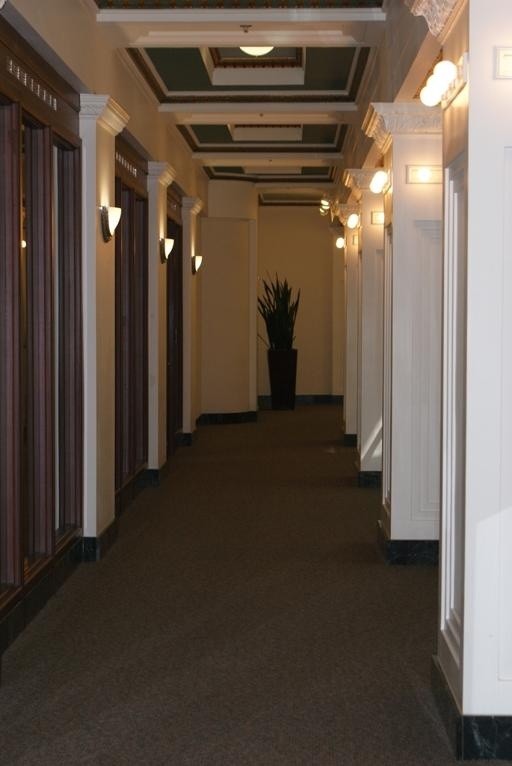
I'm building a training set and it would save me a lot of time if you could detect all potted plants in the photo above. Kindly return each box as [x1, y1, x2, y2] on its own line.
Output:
[256, 271, 302, 411]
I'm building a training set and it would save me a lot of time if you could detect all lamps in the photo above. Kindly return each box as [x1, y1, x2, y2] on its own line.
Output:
[157, 236, 174, 263]
[191, 254, 204, 273]
[100, 205, 123, 242]
[419, 50, 470, 112]
